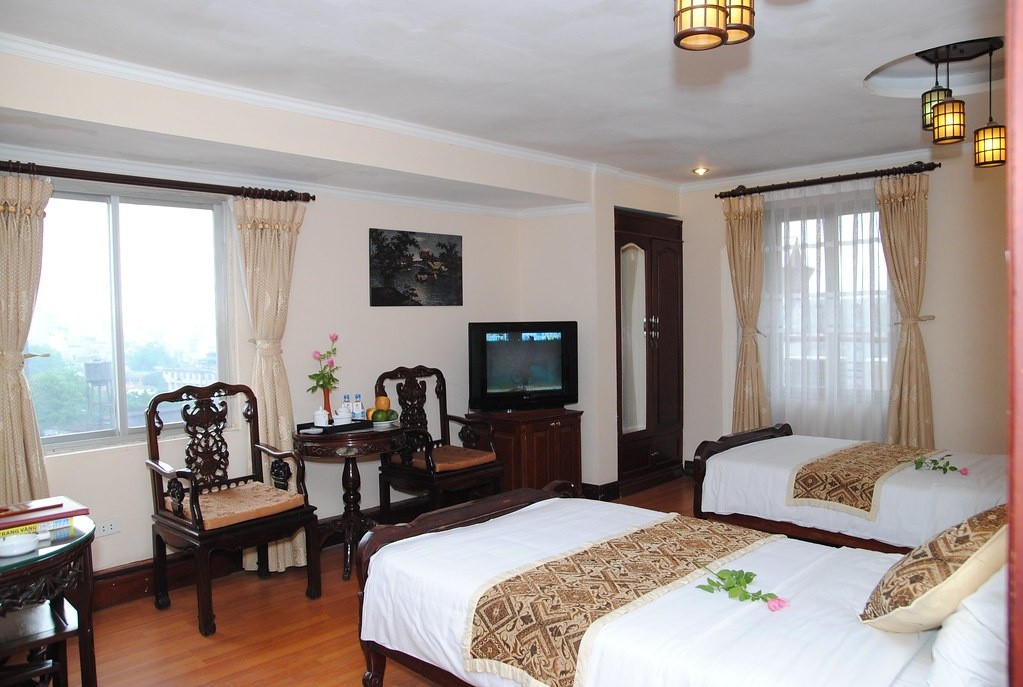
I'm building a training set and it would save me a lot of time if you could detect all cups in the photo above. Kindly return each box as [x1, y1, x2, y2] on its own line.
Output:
[332, 415, 353, 423]
[313, 410, 329, 426]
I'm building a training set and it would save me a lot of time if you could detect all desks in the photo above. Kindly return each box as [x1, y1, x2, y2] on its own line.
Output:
[351, 485, 1007, 687]
[0, 513, 100, 687]
[290, 424, 416, 580]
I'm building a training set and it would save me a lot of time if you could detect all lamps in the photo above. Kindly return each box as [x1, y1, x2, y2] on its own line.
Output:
[916, 38, 1006, 168]
[674, 0, 755, 52]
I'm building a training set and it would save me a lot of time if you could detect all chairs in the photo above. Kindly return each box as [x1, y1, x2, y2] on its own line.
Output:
[144, 382, 322, 636]
[376, 365, 504, 523]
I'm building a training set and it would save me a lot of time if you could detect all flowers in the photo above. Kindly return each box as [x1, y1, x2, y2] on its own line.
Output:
[895, 449, 970, 479]
[692, 551, 788, 612]
[307, 333, 340, 394]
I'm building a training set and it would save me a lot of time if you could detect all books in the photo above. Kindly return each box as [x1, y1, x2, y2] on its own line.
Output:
[0, 496, 90, 538]
[37, 526, 85, 550]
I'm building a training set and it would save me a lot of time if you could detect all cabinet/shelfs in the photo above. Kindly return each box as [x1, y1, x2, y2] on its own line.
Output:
[465, 409, 584, 496]
[615, 206, 684, 498]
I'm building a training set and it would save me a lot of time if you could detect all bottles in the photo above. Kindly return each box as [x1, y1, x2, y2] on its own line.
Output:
[341, 394, 353, 416]
[352, 394, 366, 419]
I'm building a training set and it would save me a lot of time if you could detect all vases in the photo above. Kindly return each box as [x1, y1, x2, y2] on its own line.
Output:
[323, 387, 334, 424]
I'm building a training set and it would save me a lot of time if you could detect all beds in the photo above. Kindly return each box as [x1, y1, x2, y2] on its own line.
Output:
[693, 424, 1010, 523]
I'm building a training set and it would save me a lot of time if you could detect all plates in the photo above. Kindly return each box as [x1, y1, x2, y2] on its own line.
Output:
[373, 420, 398, 426]
[320, 425, 333, 427]
[332, 421, 361, 426]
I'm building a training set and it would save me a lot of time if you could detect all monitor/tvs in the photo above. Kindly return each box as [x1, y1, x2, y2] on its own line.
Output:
[468, 321, 578, 413]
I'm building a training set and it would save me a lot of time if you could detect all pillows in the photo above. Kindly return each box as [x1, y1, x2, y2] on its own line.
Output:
[857, 501, 1009, 633]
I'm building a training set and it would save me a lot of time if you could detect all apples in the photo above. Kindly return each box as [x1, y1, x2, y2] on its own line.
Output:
[366, 396, 398, 422]
